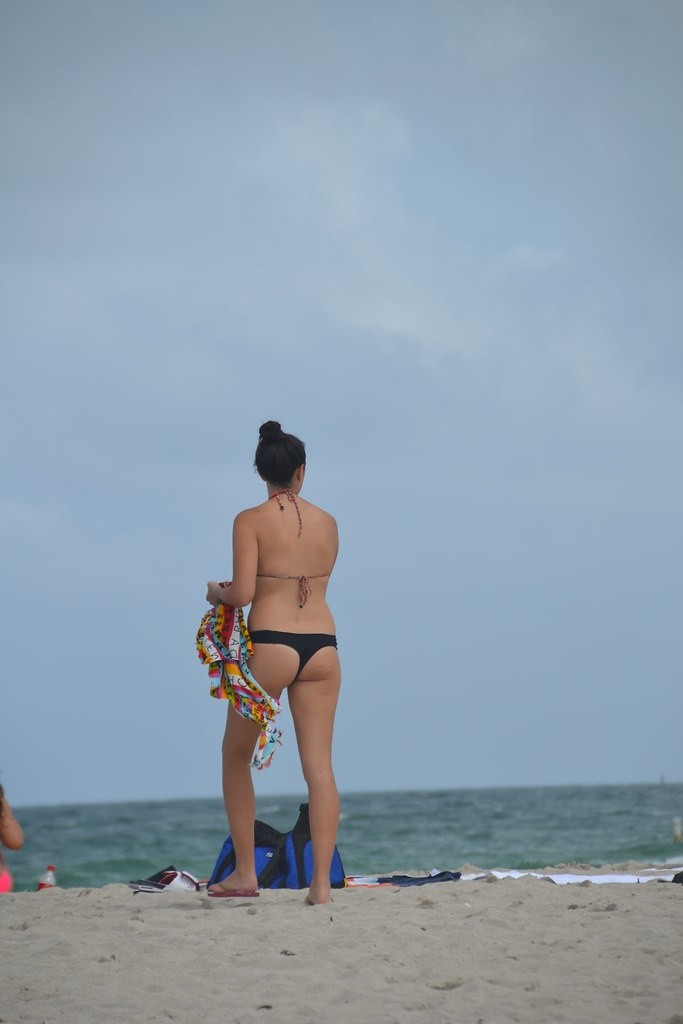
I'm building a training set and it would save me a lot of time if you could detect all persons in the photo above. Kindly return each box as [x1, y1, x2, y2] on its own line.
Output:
[206, 422, 342, 905]
[0, 785, 23, 892]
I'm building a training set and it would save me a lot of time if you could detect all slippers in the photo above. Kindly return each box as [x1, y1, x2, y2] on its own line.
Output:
[207, 882, 260, 897]
[306, 895, 334, 906]
[128, 864, 201, 893]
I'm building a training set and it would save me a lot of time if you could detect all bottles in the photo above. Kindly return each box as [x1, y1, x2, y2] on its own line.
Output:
[36, 865, 56, 891]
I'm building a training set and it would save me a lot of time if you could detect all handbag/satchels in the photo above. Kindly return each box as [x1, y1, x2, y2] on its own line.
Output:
[207, 802, 347, 889]
